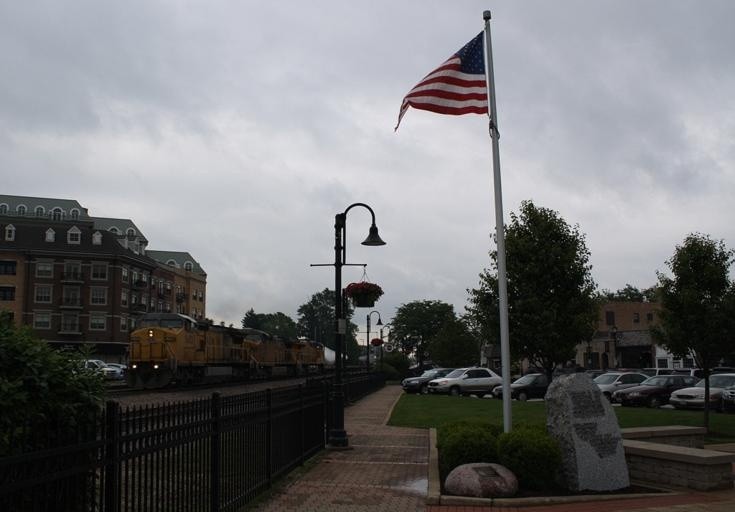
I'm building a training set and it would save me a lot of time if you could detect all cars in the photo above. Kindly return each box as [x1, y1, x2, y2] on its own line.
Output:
[70, 358, 127, 382]
[588, 367, 734, 410]
[401, 360, 548, 400]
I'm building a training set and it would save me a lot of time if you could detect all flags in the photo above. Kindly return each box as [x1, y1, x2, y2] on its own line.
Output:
[393, 25, 493, 137]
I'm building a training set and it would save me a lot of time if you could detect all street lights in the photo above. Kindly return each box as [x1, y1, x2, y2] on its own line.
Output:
[329, 204, 386, 446]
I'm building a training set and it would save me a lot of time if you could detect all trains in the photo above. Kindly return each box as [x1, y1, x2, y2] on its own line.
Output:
[128, 312, 325, 391]
